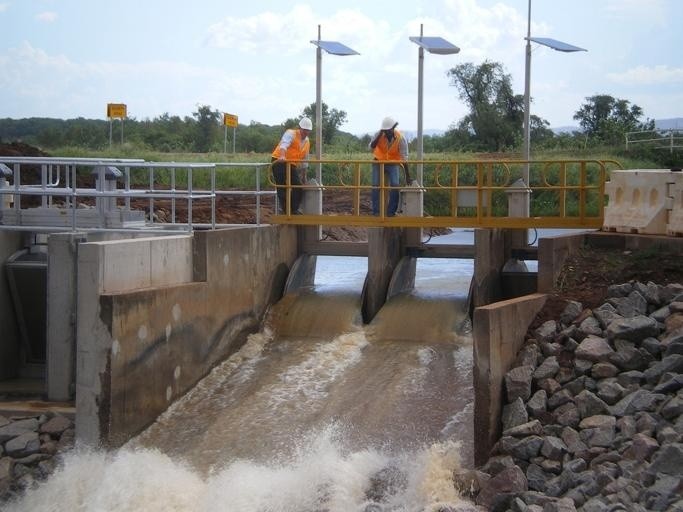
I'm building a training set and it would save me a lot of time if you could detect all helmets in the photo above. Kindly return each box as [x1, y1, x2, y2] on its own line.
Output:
[380, 117, 394, 130]
[299, 117, 312, 130]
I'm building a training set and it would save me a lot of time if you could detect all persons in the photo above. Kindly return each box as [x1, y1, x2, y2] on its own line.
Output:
[367, 116, 411, 216]
[270, 117, 312, 214]
